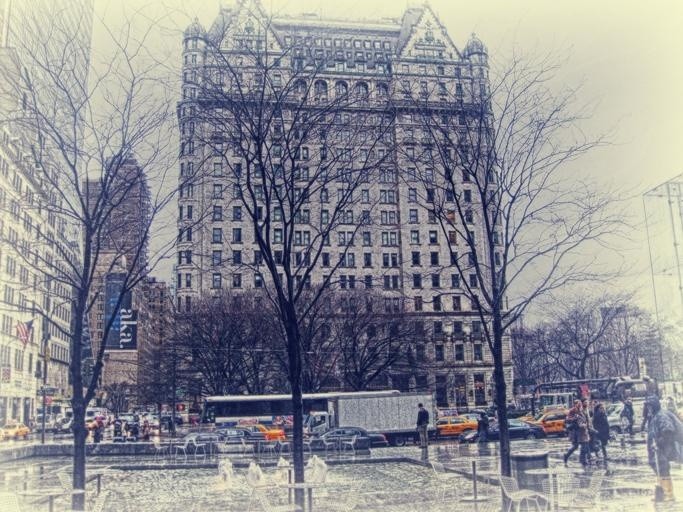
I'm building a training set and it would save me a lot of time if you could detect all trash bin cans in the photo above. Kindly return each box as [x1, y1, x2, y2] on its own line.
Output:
[509, 450, 550, 491]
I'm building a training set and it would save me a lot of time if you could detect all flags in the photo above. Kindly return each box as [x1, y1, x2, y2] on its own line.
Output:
[14, 319, 33, 344]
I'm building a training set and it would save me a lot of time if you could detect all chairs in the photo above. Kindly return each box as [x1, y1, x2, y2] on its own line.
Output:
[0, 427, 358, 512]
[421, 460, 607, 512]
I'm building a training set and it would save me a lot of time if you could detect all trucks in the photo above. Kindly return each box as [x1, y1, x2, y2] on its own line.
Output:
[302, 392, 436, 446]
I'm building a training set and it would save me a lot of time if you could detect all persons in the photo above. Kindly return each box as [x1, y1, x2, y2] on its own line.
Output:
[618, 396, 638, 448]
[93, 426, 101, 442]
[578, 396, 598, 465]
[416, 402, 429, 448]
[562, 398, 590, 466]
[643, 389, 663, 429]
[646, 408, 683, 500]
[142, 419, 149, 440]
[639, 400, 652, 433]
[119, 420, 128, 443]
[477, 414, 490, 442]
[167, 415, 173, 433]
[591, 403, 612, 460]
[96, 421, 104, 431]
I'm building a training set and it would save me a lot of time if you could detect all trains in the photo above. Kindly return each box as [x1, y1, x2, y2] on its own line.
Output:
[199, 390, 401, 432]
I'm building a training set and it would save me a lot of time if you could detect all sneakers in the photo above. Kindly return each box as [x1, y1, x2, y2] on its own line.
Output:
[564, 454, 610, 466]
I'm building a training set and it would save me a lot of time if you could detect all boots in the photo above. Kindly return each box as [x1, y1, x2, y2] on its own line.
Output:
[656, 477, 675, 502]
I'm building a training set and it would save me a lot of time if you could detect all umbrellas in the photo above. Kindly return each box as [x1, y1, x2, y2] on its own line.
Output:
[92, 415, 106, 421]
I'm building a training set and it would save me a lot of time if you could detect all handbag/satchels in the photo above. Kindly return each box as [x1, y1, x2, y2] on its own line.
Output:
[564, 421, 579, 430]
[620, 417, 629, 429]
[660, 410, 682, 466]
[588, 433, 602, 451]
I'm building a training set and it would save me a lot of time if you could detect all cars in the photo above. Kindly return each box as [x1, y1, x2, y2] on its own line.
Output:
[35, 407, 184, 440]
[315, 426, 389, 451]
[1, 423, 30, 441]
[186, 424, 315, 451]
[434, 393, 683, 444]
